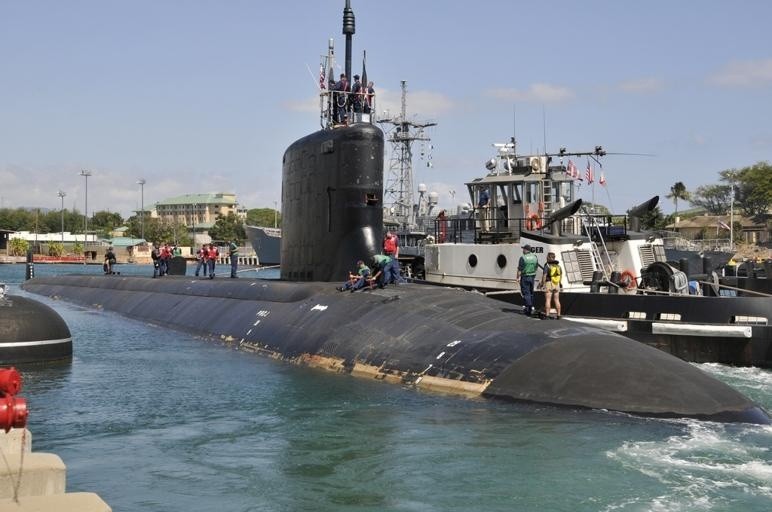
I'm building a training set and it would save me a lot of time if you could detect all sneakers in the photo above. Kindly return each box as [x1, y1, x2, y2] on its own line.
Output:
[525, 311, 561, 319]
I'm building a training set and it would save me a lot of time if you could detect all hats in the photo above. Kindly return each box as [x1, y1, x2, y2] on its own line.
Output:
[521, 245, 531, 251]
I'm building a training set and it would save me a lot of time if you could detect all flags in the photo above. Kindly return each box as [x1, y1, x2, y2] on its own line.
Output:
[361, 56, 368, 88]
[565, 160, 584, 182]
[585, 161, 595, 186]
[319, 63, 326, 89]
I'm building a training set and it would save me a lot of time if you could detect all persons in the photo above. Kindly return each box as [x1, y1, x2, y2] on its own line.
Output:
[336, 259, 371, 293]
[367, 255, 394, 290]
[327, 73, 375, 124]
[151, 238, 241, 279]
[473, 185, 491, 233]
[538, 251, 562, 321]
[105, 249, 110, 275]
[381, 229, 400, 284]
[105, 247, 116, 274]
[515, 243, 537, 315]
